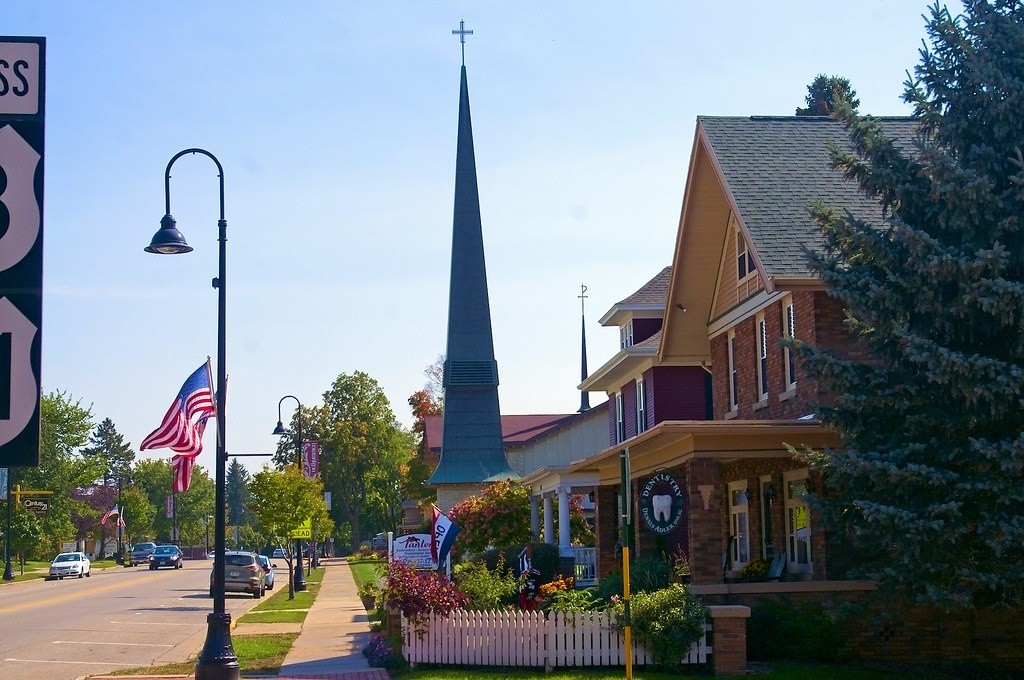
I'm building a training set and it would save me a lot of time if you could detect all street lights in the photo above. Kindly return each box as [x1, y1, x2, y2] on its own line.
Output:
[270, 394, 307, 591]
[144, 147, 242, 680]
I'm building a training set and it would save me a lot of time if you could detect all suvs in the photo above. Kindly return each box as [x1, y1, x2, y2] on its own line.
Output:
[128, 542, 157, 566]
[209, 551, 266, 598]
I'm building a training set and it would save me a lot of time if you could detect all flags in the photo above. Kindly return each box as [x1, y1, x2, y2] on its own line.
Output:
[172, 391, 216, 496]
[100, 504, 119, 525]
[140, 360, 216, 456]
[431, 504, 463, 575]
[116, 505, 127, 528]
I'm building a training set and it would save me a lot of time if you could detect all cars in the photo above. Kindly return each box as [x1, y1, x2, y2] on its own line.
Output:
[257, 555, 278, 590]
[148, 545, 183, 570]
[49, 551, 91, 581]
[273, 549, 288, 558]
[205, 548, 231, 558]
